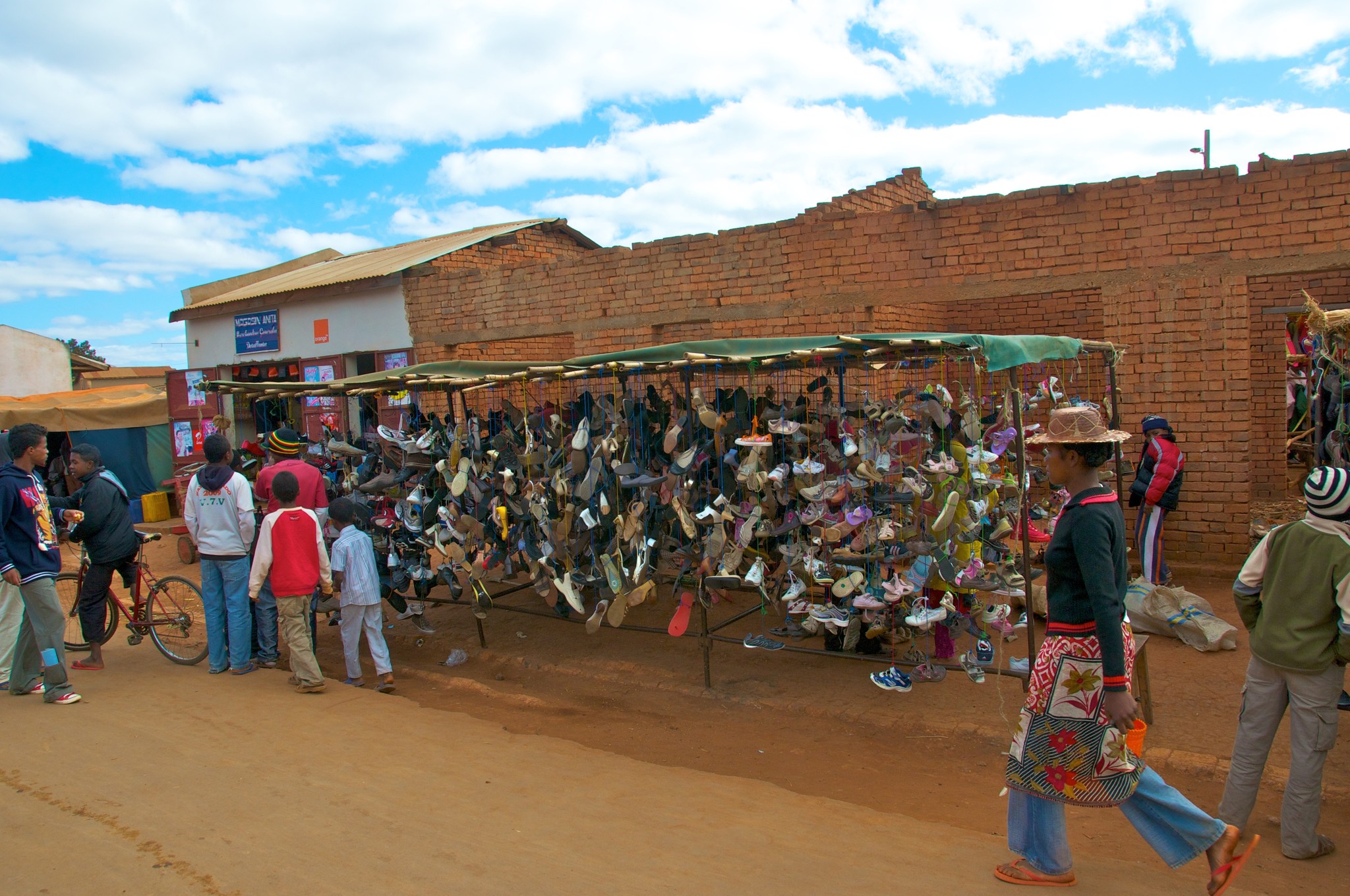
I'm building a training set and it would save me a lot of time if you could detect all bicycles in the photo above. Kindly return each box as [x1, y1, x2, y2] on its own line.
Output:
[52, 520, 208, 666]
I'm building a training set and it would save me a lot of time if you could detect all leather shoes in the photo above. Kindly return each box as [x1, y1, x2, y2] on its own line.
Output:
[1323, 373, 1346, 403]
[1324, 430, 1350, 467]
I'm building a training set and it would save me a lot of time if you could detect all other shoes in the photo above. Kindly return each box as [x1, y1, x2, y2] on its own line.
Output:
[237, 449, 257, 471]
[1282, 836, 1337, 860]
[289, 377, 1122, 692]
[242, 440, 267, 457]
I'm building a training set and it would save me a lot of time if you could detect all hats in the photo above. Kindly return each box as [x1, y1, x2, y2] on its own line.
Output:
[1022, 406, 1134, 445]
[1304, 468, 1350, 521]
[1140, 415, 1169, 433]
[261, 428, 299, 459]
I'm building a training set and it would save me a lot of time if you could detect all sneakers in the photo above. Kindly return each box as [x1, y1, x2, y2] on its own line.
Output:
[53, 693, 81, 704]
[29, 682, 46, 693]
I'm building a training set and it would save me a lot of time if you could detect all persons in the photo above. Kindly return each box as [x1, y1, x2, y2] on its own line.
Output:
[322, 376, 326, 381]
[188, 385, 195, 406]
[1217, 464, 1350, 861]
[0, 421, 87, 705]
[0, 429, 28, 692]
[204, 427, 219, 438]
[1285, 361, 1313, 469]
[994, 406, 1262, 896]
[175, 430, 187, 457]
[406, 402, 426, 437]
[191, 389, 203, 405]
[47, 452, 68, 499]
[43, 442, 146, 672]
[248, 471, 335, 695]
[1127, 412, 1186, 590]
[327, 496, 398, 695]
[222, 447, 262, 660]
[366, 412, 378, 434]
[182, 432, 259, 678]
[250, 426, 329, 672]
[385, 356, 407, 370]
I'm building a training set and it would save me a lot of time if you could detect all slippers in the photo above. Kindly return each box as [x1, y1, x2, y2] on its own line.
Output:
[994, 857, 1078, 888]
[138, 599, 147, 605]
[71, 659, 102, 670]
[40, 666, 46, 675]
[232, 662, 259, 675]
[209, 660, 230, 673]
[0, 680, 10, 690]
[250, 658, 277, 668]
[1207, 835, 1260, 895]
